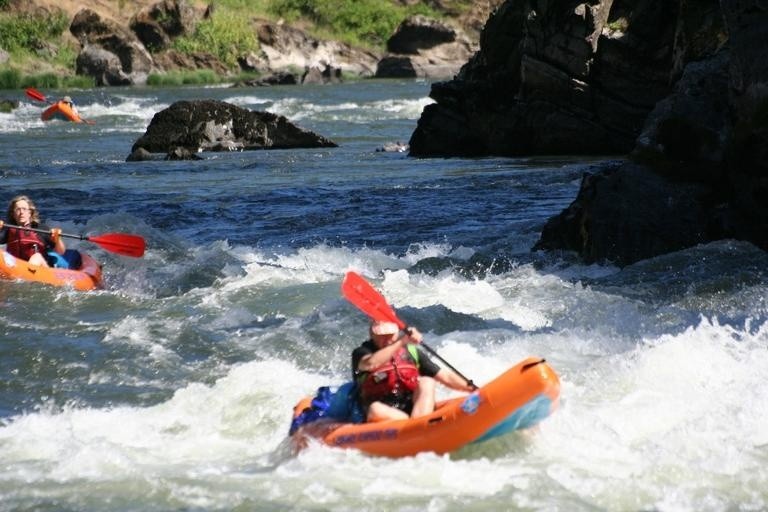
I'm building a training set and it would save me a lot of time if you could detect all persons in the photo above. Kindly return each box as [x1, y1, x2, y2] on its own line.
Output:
[0, 195, 66, 266]
[56, 95, 77, 112]
[351, 318, 478, 423]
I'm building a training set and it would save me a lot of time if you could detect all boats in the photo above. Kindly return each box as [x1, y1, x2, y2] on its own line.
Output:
[41, 100, 80, 122]
[293, 354, 561, 462]
[0, 245, 102, 293]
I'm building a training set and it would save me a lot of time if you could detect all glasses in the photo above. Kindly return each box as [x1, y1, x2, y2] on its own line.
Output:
[12, 208, 32, 212]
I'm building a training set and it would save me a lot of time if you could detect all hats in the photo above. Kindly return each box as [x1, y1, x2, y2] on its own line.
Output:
[63, 96, 71, 103]
[370, 320, 398, 336]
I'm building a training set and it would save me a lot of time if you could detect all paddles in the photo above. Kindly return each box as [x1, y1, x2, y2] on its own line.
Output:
[0, 221, 144, 257]
[25, 88, 88, 122]
[342, 271, 479, 391]
